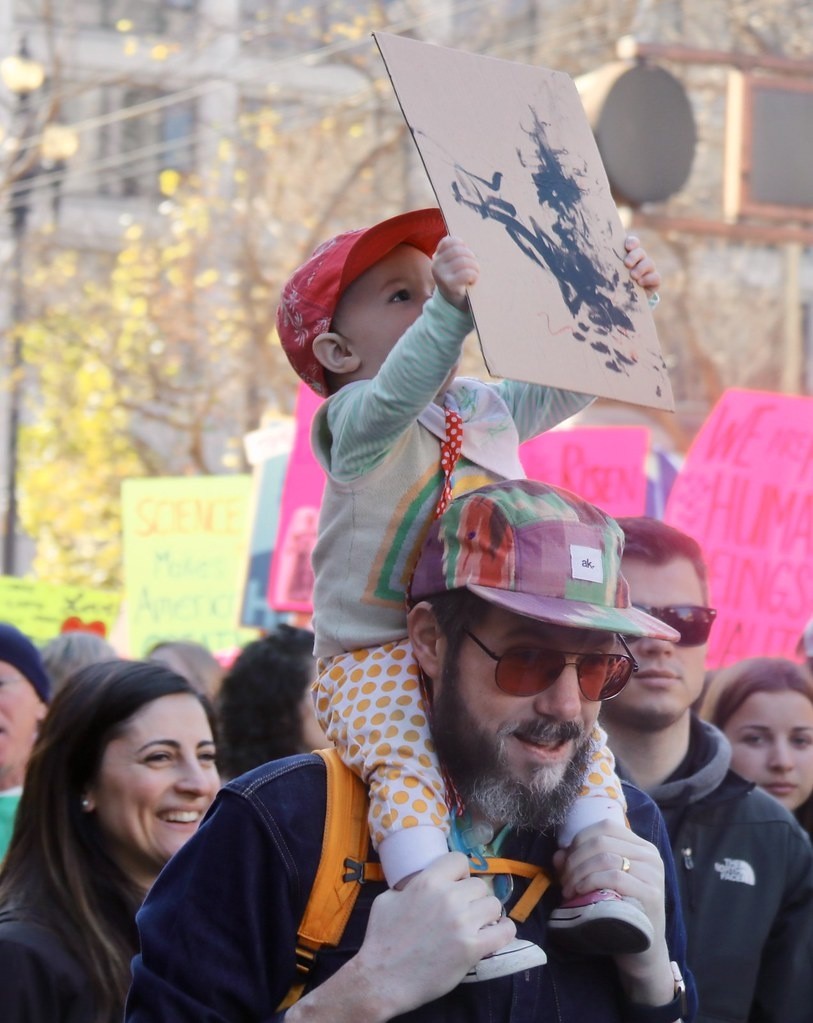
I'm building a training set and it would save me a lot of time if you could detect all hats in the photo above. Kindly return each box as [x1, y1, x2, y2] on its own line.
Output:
[275, 208, 448, 399]
[0, 623, 50, 701]
[411, 480, 681, 644]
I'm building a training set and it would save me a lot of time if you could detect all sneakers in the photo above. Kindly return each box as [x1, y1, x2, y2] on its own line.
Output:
[458, 914, 548, 984]
[548, 887, 654, 954]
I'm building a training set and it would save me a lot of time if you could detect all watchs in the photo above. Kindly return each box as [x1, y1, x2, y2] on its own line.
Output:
[626, 961, 687, 1023]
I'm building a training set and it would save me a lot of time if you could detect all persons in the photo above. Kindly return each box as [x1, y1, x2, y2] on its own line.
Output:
[44, 633, 223, 698]
[278, 208, 660, 985]
[692, 613, 813, 840]
[125, 480, 700, 1023]
[219, 628, 336, 786]
[595, 517, 813, 1023]
[0, 623, 51, 866]
[0, 659, 219, 1023]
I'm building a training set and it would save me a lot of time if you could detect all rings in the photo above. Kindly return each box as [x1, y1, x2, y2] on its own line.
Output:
[622, 858, 630, 872]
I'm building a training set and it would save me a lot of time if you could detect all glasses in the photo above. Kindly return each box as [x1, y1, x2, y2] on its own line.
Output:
[622, 603, 717, 648]
[460, 625, 639, 702]
